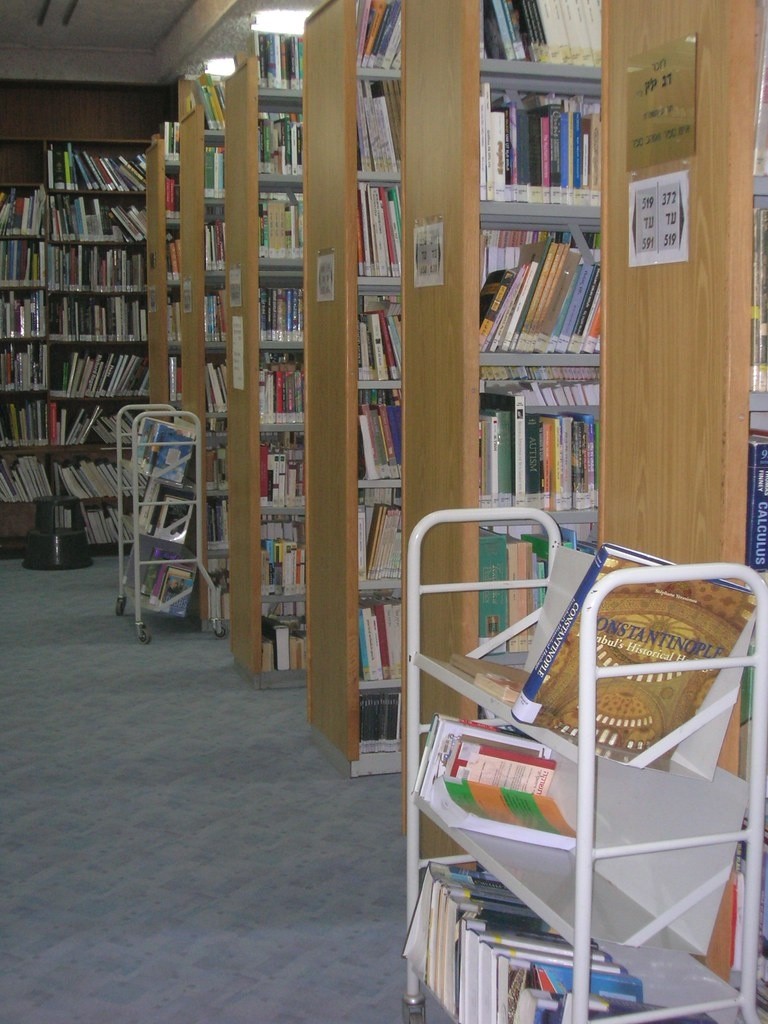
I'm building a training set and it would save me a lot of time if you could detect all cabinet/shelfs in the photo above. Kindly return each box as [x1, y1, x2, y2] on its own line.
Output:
[0, 0, 768, 1024]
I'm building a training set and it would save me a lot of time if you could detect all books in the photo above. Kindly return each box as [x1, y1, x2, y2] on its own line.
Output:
[474, 672, 522, 703]
[414, 713, 557, 801]
[430, 775, 577, 852]
[474, 1, 768, 775]
[0, 140, 151, 619]
[130, 3, 404, 776]
[508, 541, 757, 759]
[402, 860, 698, 1024]
